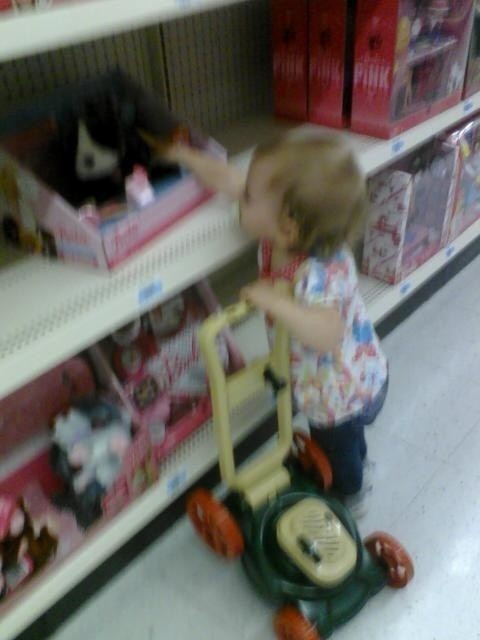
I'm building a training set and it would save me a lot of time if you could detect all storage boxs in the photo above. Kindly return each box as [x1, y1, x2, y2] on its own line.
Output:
[0, 71, 234, 269]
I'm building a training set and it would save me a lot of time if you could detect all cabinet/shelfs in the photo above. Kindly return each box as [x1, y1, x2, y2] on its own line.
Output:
[0, 2, 479, 640]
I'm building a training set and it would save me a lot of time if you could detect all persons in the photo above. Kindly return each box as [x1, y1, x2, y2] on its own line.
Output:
[154, 123, 392, 519]
